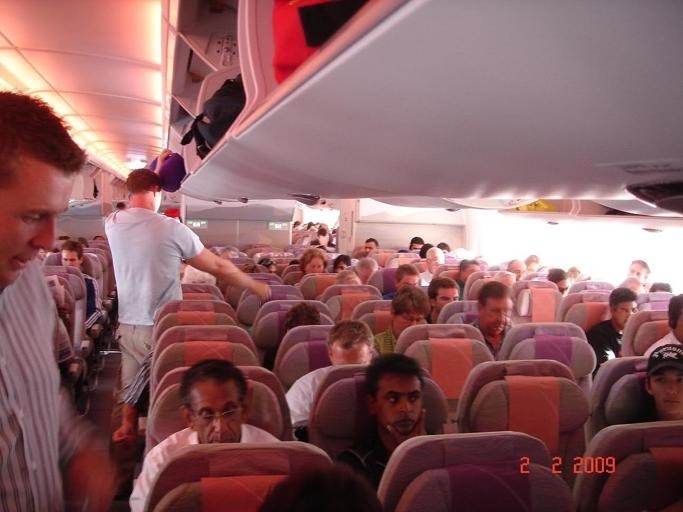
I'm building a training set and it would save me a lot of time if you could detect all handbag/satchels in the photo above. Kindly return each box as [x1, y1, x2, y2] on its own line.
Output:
[195, 74, 246, 148]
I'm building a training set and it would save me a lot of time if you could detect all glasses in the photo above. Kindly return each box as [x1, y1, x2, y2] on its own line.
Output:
[195, 410, 243, 424]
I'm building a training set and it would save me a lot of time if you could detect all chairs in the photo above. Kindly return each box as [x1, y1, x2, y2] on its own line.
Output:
[31, 239, 116, 405]
[144, 229, 683, 512]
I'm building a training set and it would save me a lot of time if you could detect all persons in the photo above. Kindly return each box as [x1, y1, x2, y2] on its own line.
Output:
[1, 90, 119, 512]
[36, 170, 683, 512]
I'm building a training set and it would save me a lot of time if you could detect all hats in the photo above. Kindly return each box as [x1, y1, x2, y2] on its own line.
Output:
[648, 345, 683, 377]
